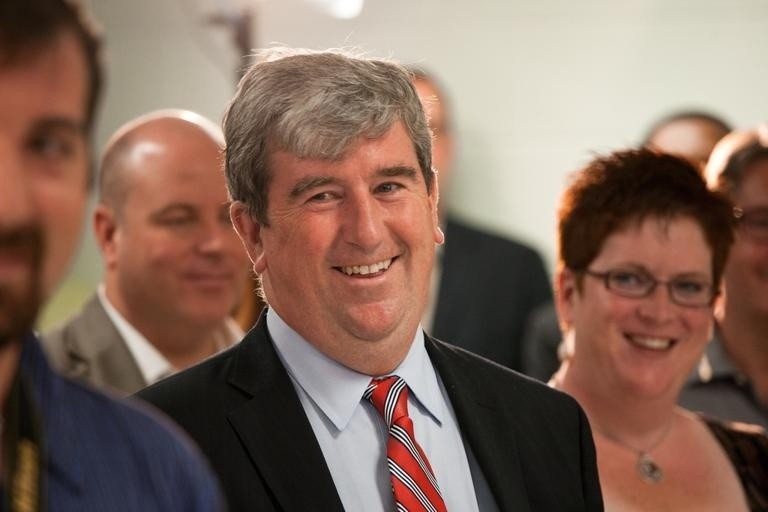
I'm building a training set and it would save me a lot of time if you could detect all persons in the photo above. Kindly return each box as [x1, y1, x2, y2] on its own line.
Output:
[248, 69, 564, 383]
[108, 50, 605, 511]
[0, 0, 229, 512]
[641, 110, 736, 382]
[39, 108, 250, 401]
[543, 146, 767, 512]
[674, 122, 766, 444]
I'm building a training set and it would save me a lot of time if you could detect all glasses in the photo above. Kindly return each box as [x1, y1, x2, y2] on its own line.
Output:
[583, 263, 721, 311]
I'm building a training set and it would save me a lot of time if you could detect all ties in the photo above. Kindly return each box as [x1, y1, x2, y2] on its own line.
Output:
[361, 375, 451, 511]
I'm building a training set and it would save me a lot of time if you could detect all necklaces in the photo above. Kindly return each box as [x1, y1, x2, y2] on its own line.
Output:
[542, 372, 681, 489]
